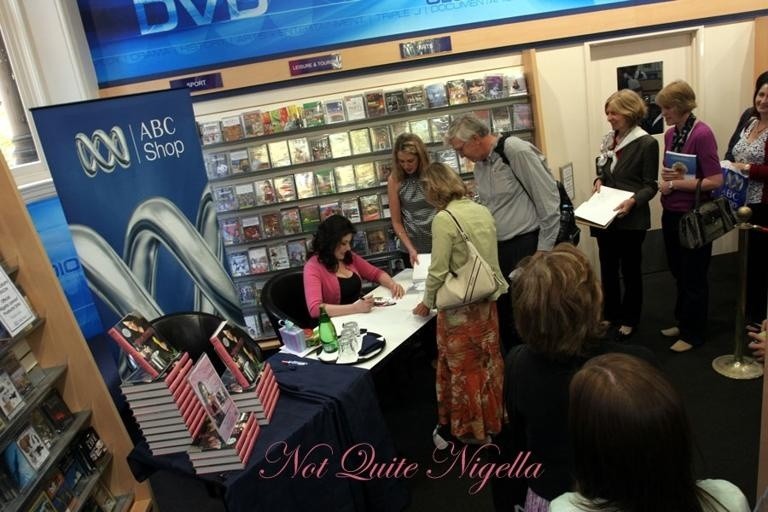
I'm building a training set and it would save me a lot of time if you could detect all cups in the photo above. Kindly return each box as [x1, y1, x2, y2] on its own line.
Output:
[340, 329, 359, 350]
[343, 321, 360, 334]
[336, 337, 357, 362]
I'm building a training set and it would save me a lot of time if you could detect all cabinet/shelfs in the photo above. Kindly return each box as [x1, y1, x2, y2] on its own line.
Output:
[191, 46, 549, 353]
[0, 150, 162, 512]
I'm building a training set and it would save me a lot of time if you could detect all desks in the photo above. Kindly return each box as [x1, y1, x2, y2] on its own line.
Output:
[127, 266, 437, 512]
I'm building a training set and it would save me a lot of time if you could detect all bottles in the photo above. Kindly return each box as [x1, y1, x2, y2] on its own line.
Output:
[319, 305, 339, 354]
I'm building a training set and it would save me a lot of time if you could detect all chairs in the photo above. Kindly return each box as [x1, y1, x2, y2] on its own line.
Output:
[260, 268, 321, 347]
[119, 310, 226, 381]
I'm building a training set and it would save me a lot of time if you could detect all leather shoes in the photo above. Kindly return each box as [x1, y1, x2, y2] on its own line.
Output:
[660, 326, 678, 336]
[613, 327, 633, 341]
[670, 340, 692, 352]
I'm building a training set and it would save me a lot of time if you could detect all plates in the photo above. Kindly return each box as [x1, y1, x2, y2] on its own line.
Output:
[318, 332, 386, 365]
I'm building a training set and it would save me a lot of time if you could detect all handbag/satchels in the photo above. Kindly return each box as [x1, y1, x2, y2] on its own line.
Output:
[680, 179, 740, 253]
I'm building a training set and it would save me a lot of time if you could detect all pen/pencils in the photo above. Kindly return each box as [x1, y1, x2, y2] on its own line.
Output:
[359, 296, 380, 307]
[282, 360, 307, 366]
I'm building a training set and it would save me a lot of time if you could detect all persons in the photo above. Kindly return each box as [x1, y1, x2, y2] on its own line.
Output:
[387, 133, 441, 268]
[413, 163, 511, 446]
[746, 319, 766, 363]
[303, 215, 404, 329]
[723, 72, 768, 162]
[732, 80, 768, 331]
[655, 81, 739, 355]
[592, 89, 659, 343]
[548, 352, 753, 511]
[446, 112, 561, 353]
[486, 242, 644, 512]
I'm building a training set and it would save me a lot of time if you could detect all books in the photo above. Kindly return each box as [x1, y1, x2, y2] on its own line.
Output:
[718, 162, 750, 206]
[117, 353, 207, 455]
[384, 69, 534, 169]
[223, 363, 281, 425]
[200, 88, 396, 222]
[662, 150, 697, 182]
[217, 200, 303, 340]
[108, 310, 181, 378]
[1, 427, 118, 511]
[573, 184, 634, 228]
[187, 413, 260, 476]
[1, 341, 75, 432]
[304, 220, 403, 256]
[210, 319, 264, 389]
[186, 351, 241, 445]
